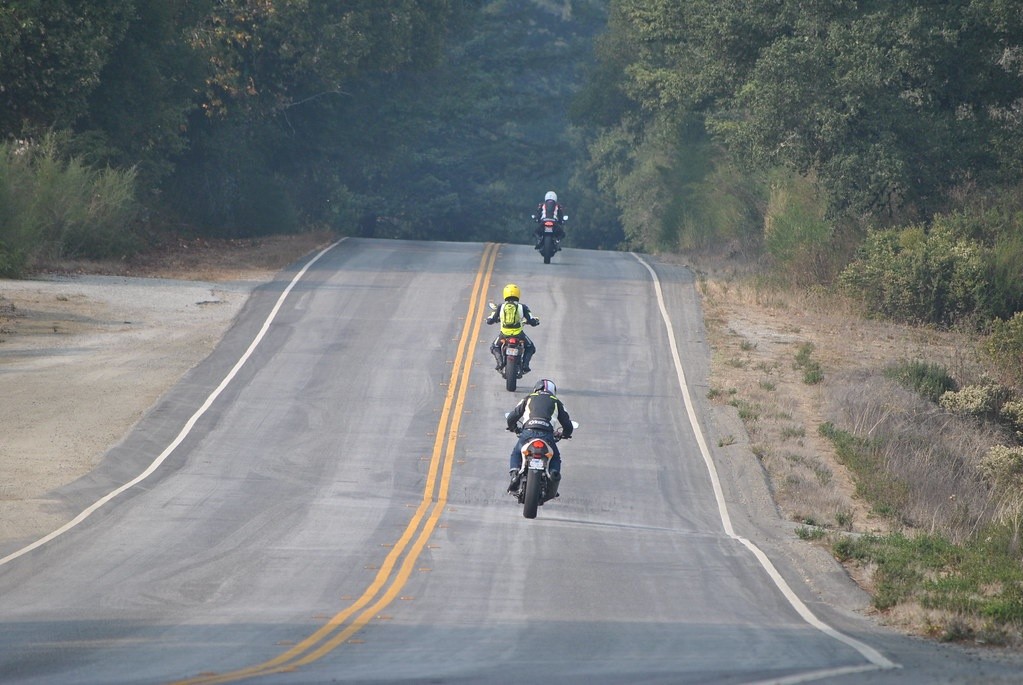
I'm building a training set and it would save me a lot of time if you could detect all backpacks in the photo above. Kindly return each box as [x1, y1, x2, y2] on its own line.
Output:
[502, 301, 523, 329]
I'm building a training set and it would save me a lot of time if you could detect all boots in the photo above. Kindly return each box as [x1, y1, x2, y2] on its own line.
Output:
[522, 347, 532, 372]
[534, 233, 542, 249]
[556, 235, 565, 250]
[493, 348, 503, 370]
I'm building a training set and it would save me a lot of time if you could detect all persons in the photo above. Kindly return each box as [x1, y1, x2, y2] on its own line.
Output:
[507, 379, 573, 497]
[486, 284, 539, 371]
[533, 191, 566, 250]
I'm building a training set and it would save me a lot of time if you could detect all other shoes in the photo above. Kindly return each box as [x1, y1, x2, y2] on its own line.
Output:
[555, 492, 560, 497]
[506, 471, 521, 493]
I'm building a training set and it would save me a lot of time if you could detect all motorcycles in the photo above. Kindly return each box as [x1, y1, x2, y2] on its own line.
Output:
[531, 215, 569, 263]
[485, 301, 540, 391]
[504, 412, 579, 518]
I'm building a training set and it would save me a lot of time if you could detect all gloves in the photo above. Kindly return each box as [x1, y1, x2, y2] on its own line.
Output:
[512, 424, 521, 434]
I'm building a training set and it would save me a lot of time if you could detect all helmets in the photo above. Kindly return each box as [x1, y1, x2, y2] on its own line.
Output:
[533, 379, 557, 397]
[545, 191, 558, 202]
[503, 283, 521, 300]
